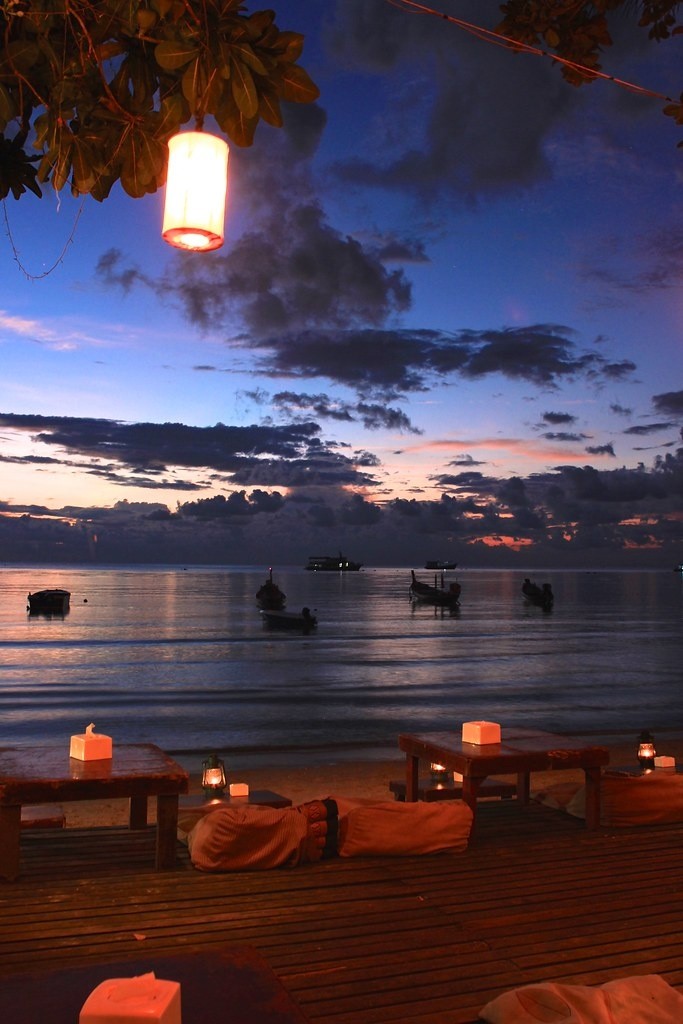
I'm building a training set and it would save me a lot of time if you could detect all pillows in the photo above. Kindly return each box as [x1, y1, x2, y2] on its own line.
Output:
[478, 975, 683, 1024]
[191, 806, 311, 872]
[336, 802, 473, 857]
[567, 773, 683, 828]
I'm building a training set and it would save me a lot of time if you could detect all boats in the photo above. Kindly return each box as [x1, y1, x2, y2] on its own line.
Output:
[256, 568, 286, 607]
[521, 578, 553, 606]
[408, 569, 461, 607]
[25, 588, 71, 616]
[424, 560, 458, 569]
[304, 551, 364, 572]
[258, 608, 316, 625]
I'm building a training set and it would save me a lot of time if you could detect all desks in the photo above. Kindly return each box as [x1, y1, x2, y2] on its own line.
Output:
[388, 778, 518, 802]
[178, 790, 293, 809]
[20, 805, 67, 830]
[398, 727, 610, 831]
[604, 763, 683, 778]
[0, 945, 311, 1024]
[0, 742, 190, 883]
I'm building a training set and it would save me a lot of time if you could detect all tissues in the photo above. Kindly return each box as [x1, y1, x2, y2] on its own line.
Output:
[461, 721, 501, 746]
[69, 723, 112, 763]
[654, 756, 675, 768]
[79, 971, 181, 1023]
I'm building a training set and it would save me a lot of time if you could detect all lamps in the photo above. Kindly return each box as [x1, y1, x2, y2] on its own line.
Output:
[636, 728, 656, 770]
[429, 762, 450, 784]
[200, 751, 228, 800]
[161, 114, 231, 252]
